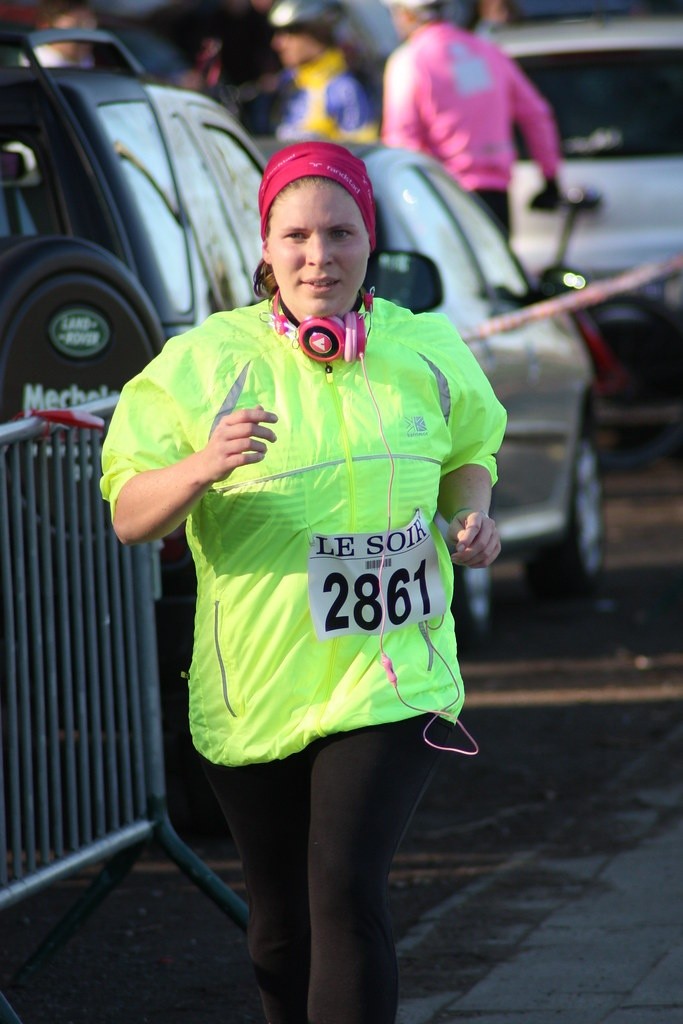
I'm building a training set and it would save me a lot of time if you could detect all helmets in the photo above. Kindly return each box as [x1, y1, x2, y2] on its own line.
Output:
[268, 0, 345, 36]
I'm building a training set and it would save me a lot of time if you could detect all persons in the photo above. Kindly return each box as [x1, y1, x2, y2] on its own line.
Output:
[99, 141, 507, 1024]
[267, 0, 381, 160]
[16, 0, 96, 69]
[381, 1, 566, 245]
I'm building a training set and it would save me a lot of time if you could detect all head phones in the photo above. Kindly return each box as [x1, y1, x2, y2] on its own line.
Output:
[270, 285, 374, 363]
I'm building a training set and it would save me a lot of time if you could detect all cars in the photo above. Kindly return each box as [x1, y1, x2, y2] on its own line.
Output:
[483, 13, 683, 394]
[256, 132, 613, 647]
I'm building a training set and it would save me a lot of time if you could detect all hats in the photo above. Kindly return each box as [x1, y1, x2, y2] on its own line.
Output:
[260, 142, 376, 248]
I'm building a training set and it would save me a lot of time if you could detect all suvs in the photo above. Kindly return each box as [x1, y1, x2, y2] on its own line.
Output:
[1, 22, 442, 659]
